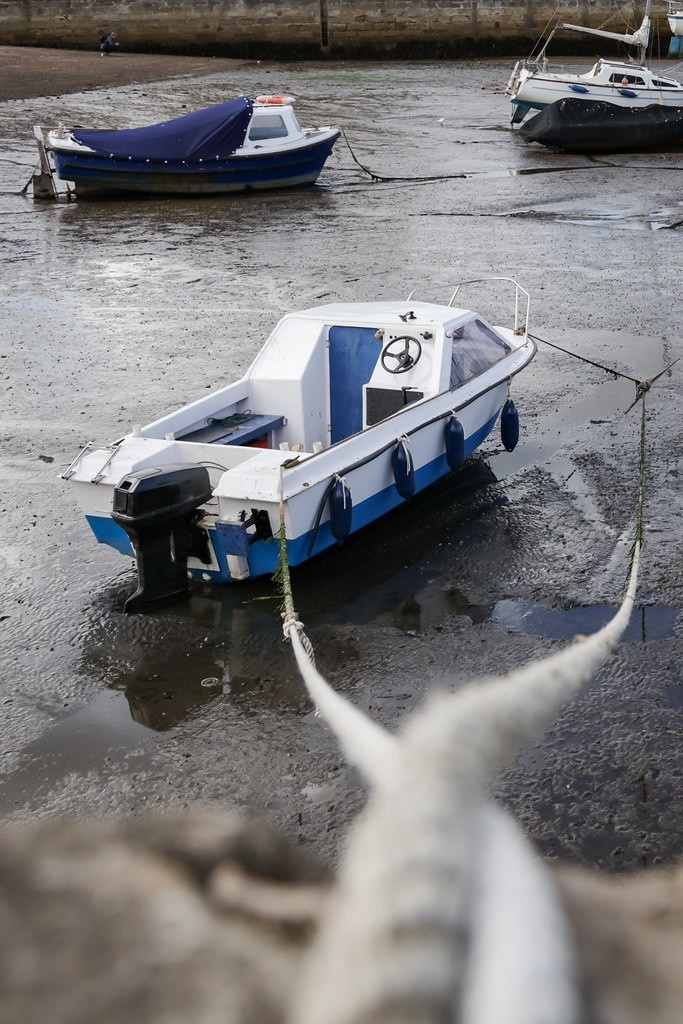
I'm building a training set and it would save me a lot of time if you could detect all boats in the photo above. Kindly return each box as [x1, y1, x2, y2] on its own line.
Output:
[52, 277, 540, 618]
[666, 10, 683, 37]
[518, 98, 683, 153]
[501, 13, 683, 111]
[34, 91, 342, 206]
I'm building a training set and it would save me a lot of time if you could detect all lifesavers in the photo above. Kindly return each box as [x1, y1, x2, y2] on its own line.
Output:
[255, 94, 295, 105]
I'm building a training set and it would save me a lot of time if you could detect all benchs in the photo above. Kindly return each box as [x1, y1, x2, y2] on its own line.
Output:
[176, 414, 287, 446]
